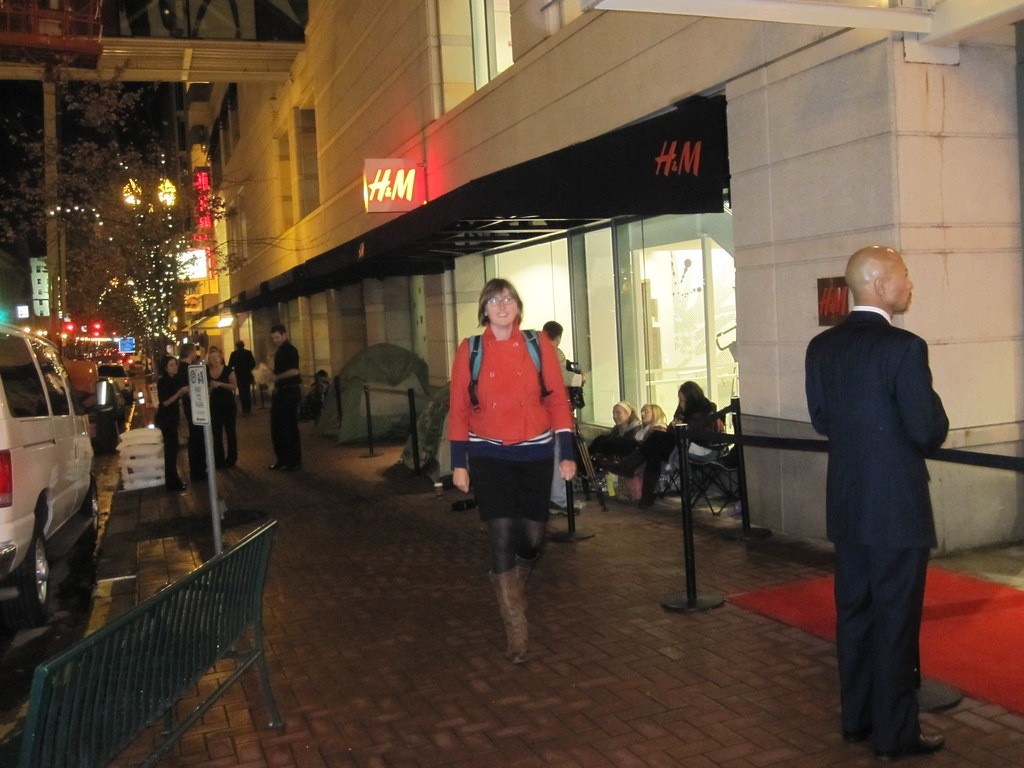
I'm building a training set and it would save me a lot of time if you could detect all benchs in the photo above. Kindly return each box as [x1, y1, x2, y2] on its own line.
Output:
[0, 517, 288, 768]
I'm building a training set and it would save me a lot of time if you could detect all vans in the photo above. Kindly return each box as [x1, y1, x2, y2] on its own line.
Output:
[0, 323, 110, 627]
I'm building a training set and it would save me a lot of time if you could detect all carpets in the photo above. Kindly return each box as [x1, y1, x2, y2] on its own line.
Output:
[726, 565, 1024, 720]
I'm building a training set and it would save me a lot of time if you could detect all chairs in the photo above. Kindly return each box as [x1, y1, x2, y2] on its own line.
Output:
[660, 414, 742, 517]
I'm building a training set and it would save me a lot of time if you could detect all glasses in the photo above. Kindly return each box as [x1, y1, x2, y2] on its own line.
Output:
[487, 295, 515, 307]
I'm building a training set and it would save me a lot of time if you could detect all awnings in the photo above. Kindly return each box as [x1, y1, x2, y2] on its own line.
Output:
[192, 93, 726, 321]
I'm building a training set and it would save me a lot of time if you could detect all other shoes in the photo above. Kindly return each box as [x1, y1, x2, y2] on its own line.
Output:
[268, 462, 302, 471]
[638, 498, 654, 509]
[173, 483, 186, 489]
[611, 455, 628, 470]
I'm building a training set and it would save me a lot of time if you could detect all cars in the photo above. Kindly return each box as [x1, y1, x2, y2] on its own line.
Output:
[72, 376, 127, 456]
[96, 363, 133, 404]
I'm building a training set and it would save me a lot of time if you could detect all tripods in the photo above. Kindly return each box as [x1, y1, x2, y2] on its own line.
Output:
[568, 405, 610, 513]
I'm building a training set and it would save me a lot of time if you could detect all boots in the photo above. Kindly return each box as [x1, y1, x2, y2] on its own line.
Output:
[488, 567, 529, 663]
[515, 551, 542, 604]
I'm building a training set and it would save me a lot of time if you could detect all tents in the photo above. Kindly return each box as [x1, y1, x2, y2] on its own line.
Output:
[400, 381, 469, 480]
[315, 343, 429, 444]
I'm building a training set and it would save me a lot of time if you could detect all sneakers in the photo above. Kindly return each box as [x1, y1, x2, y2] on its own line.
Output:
[548, 501, 581, 516]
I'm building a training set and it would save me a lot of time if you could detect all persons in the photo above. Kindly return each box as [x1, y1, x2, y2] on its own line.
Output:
[178, 343, 208, 480]
[541, 321, 580, 517]
[448, 279, 577, 665]
[608, 381, 724, 509]
[588, 402, 667, 473]
[155, 356, 189, 491]
[205, 346, 238, 470]
[269, 325, 303, 474]
[228, 341, 256, 412]
[805, 245, 949, 763]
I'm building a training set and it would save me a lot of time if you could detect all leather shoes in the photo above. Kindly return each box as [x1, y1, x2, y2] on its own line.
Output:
[875, 733, 944, 762]
[843, 725, 871, 742]
